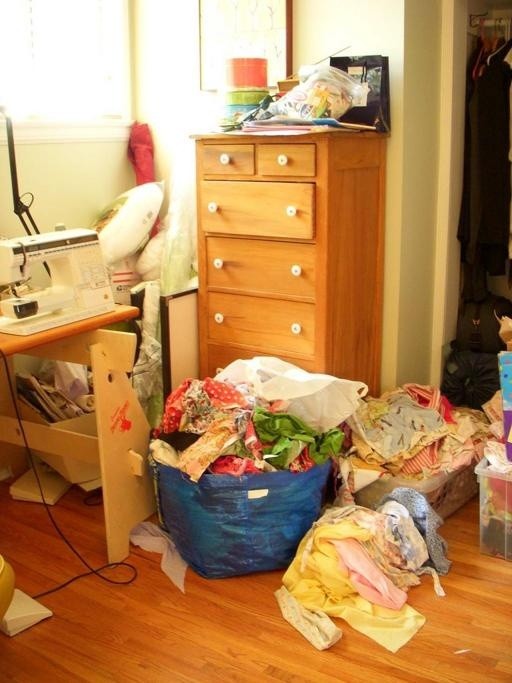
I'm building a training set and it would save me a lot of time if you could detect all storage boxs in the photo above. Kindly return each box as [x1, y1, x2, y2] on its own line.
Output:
[354, 437, 512, 563]
[14, 396, 104, 485]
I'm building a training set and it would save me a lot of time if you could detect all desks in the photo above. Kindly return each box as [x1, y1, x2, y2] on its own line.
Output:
[0, 302, 160, 570]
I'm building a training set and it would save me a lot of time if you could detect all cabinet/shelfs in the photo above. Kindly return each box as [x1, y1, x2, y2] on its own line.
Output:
[188, 129, 392, 456]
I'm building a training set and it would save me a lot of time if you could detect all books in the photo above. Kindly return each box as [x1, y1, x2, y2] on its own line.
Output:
[12, 367, 87, 423]
[8, 465, 71, 505]
[0, 586, 54, 638]
[277, 77, 300, 90]
[242, 116, 377, 133]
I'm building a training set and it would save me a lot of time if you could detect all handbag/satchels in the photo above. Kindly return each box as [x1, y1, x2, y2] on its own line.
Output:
[329, 54, 389, 131]
[455, 293, 511, 352]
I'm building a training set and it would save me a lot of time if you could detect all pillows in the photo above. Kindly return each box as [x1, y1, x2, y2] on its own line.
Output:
[87, 178, 166, 265]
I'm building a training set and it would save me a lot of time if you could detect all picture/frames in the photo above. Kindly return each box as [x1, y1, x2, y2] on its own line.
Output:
[159, 288, 198, 413]
